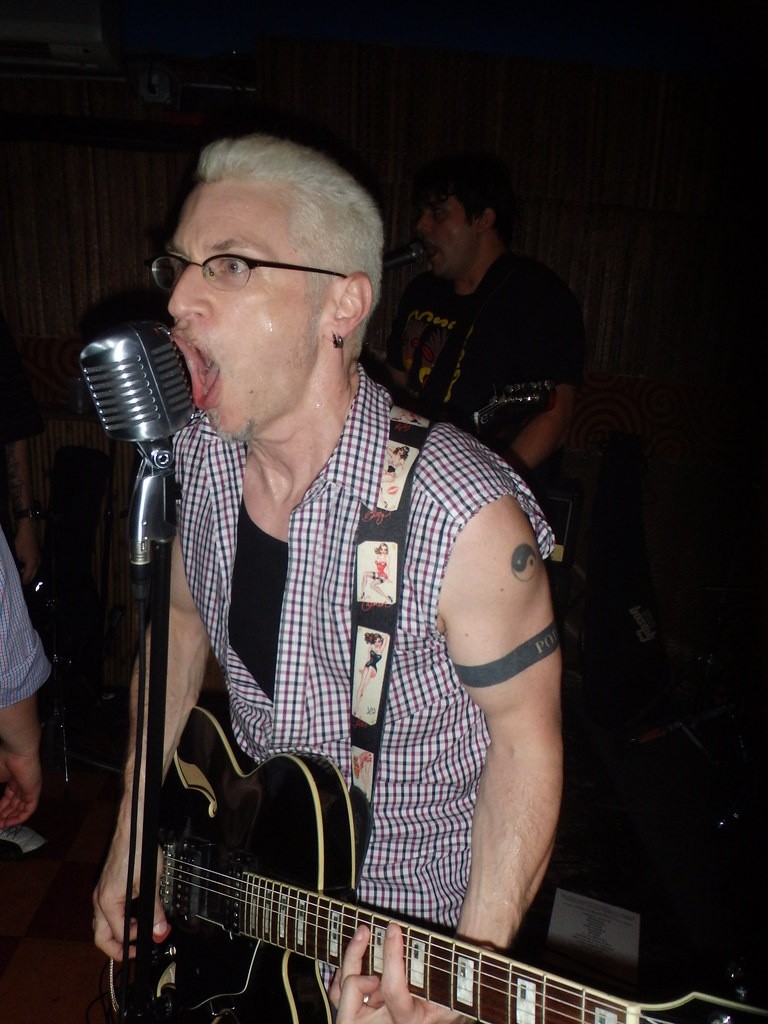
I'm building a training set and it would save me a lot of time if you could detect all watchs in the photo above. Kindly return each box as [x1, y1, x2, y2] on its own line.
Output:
[14, 501, 42, 521]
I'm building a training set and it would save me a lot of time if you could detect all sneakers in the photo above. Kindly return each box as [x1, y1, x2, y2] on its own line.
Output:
[0, 825, 50, 861]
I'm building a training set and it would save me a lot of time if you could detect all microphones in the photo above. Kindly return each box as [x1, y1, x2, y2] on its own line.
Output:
[383, 239, 428, 271]
[78, 320, 195, 444]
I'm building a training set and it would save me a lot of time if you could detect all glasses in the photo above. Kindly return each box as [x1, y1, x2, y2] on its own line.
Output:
[149, 249, 354, 292]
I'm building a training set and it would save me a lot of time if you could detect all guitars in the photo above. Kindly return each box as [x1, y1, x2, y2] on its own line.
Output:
[450, 379, 558, 437]
[102, 686, 768, 1024]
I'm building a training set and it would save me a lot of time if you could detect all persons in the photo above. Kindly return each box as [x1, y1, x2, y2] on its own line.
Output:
[1, 525, 53, 828]
[373, 152, 586, 476]
[93, 134, 563, 1024]
[0, 308, 43, 584]
